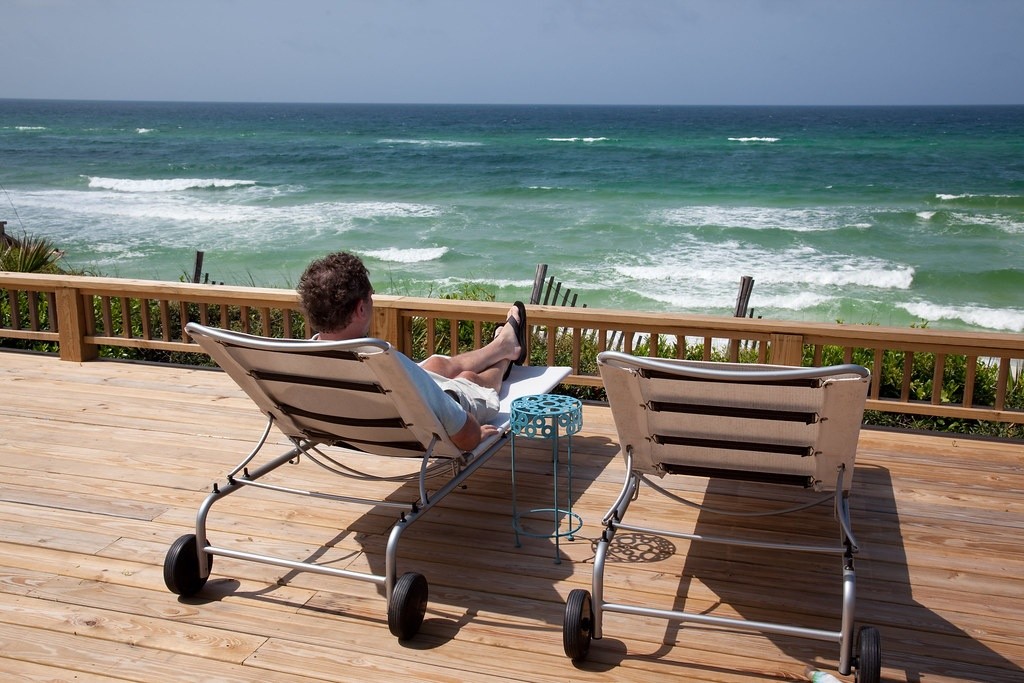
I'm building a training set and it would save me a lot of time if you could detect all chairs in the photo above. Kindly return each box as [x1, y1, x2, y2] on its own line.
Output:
[563, 351, 881, 683]
[164, 322, 572, 638]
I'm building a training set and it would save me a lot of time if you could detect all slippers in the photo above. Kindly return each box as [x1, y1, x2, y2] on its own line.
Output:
[493, 323, 513, 381]
[506, 301, 527, 366]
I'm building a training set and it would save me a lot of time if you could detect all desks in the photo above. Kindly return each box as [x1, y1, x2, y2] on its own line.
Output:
[510, 394, 583, 564]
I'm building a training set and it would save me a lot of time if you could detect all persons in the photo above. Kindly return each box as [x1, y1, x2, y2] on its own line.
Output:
[297, 251, 527, 450]
